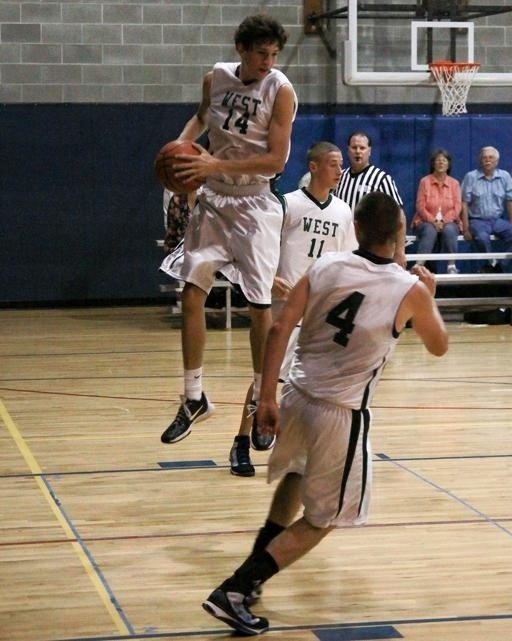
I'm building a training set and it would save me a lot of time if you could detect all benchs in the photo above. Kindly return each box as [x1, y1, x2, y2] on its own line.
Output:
[156, 233, 512, 329]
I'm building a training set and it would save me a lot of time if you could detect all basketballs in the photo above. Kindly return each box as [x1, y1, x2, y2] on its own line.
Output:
[155, 140, 204, 193]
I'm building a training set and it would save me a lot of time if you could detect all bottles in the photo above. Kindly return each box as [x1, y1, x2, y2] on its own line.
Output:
[434, 206, 444, 226]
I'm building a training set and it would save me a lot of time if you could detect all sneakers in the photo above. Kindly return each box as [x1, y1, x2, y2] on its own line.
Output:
[447, 264, 458, 274]
[491, 263, 504, 273]
[200, 578, 269, 636]
[250, 399, 277, 451]
[480, 264, 494, 273]
[160, 390, 216, 444]
[228, 435, 256, 477]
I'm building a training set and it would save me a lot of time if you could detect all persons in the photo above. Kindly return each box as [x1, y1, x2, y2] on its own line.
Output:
[157, 15, 299, 452]
[330, 131, 408, 267]
[163, 193, 247, 309]
[229, 140, 357, 476]
[409, 148, 463, 274]
[202, 193, 450, 635]
[461, 146, 512, 273]
